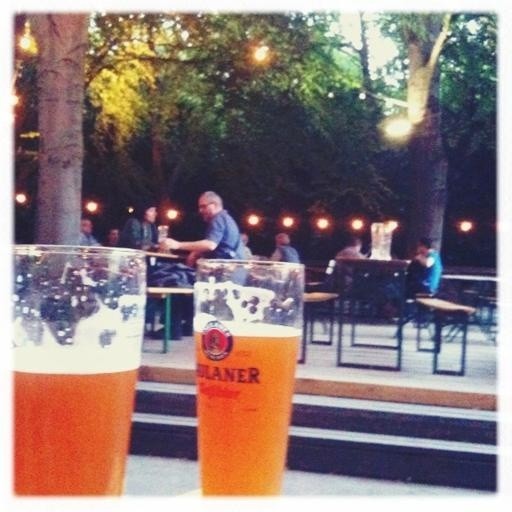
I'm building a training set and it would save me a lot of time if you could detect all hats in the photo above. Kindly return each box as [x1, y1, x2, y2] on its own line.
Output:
[421, 236, 437, 249]
[134, 196, 161, 215]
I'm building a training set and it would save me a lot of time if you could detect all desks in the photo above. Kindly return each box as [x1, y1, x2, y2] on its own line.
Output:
[332, 255, 411, 373]
[140, 249, 179, 261]
[442, 273, 497, 344]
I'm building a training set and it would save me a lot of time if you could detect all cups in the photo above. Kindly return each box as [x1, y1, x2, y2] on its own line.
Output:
[191, 254, 308, 496]
[13, 241, 148, 495]
[369, 222, 394, 261]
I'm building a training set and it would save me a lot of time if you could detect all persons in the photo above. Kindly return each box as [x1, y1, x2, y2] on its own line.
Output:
[78, 219, 100, 245]
[239, 232, 253, 258]
[410, 237, 443, 296]
[120, 201, 164, 338]
[165, 190, 244, 267]
[102, 225, 121, 247]
[334, 238, 367, 257]
[270, 233, 300, 264]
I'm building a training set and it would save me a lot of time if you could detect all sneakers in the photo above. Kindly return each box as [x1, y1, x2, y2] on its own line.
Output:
[151, 321, 192, 341]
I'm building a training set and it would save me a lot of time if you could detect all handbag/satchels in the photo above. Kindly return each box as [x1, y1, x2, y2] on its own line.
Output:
[149, 260, 197, 286]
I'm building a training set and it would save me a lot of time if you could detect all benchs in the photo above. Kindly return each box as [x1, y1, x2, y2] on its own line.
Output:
[414, 297, 475, 377]
[298, 291, 338, 364]
[146, 285, 195, 353]
